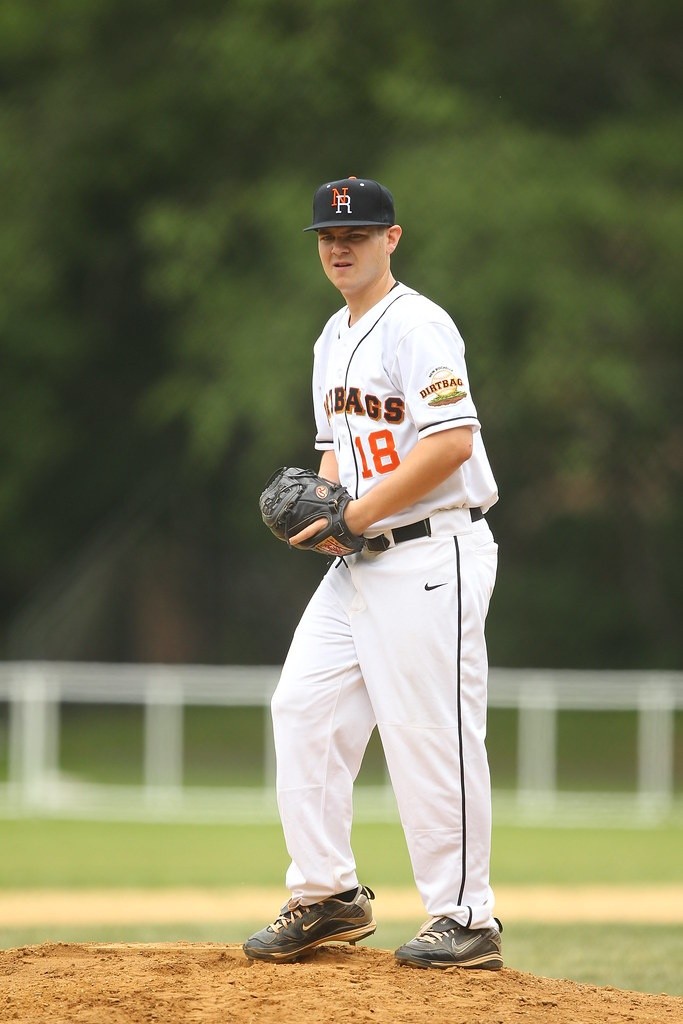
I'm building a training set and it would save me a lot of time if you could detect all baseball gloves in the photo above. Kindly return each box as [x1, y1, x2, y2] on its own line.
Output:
[256, 463, 368, 558]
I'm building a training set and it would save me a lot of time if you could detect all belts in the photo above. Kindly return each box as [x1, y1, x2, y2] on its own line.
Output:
[362, 506, 484, 551]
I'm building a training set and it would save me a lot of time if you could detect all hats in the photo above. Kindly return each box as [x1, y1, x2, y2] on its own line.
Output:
[302, 176, 395, 234]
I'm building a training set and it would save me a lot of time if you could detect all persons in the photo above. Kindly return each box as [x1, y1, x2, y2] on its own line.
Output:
[243, 176, 504, 971]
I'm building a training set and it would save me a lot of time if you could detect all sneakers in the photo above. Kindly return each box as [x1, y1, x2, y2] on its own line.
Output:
[394, 915, 504, 970]
[242, 882, 378, 961]
